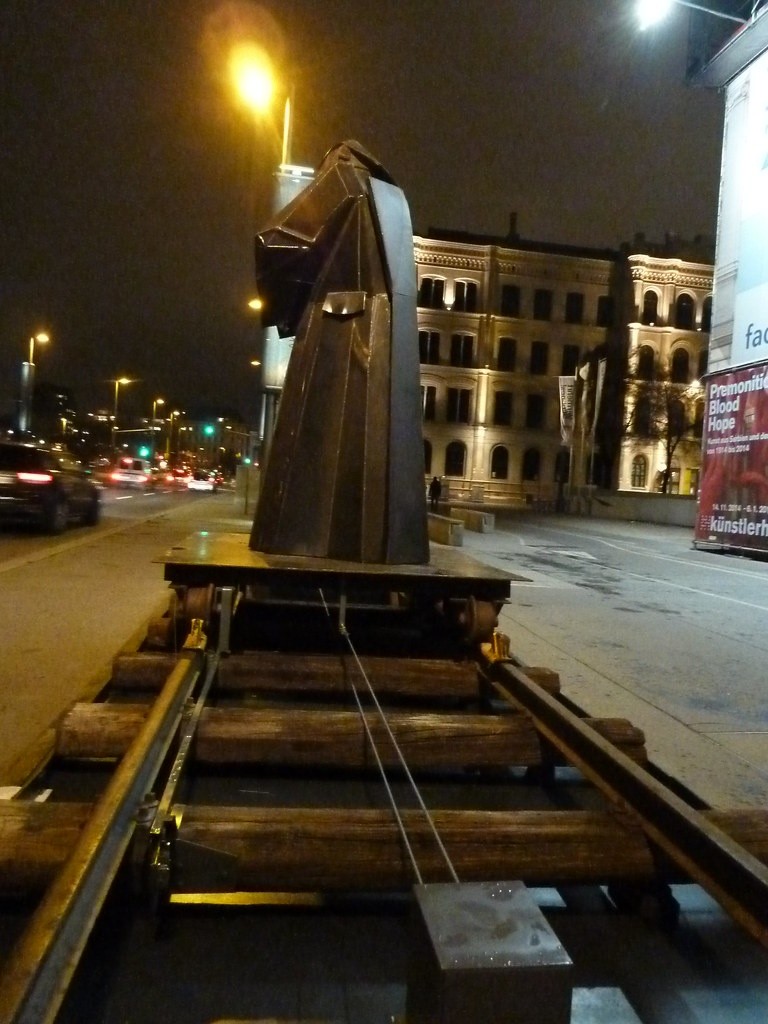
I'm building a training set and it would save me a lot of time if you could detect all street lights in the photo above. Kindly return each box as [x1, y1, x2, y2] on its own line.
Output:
[24, 331, 51, 432]
[166, 410, 180, 456]
[225, 43, 299, 171]
[149, 398, 164, 457]
[111, 374, 130, 447]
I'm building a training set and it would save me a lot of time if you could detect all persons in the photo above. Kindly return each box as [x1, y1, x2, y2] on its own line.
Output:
[429, 477, 441, 512]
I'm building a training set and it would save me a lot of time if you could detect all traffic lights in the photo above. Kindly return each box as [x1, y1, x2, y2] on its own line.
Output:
[205, 426, 216, 434]
[139, 447, 149, 457]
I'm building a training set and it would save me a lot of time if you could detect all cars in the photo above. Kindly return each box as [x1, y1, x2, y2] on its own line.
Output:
[168, 468, 189, 486]
[0, 436, 103, 537]
[188, 470, 217, 492]
[109, 457, 156, 490]
